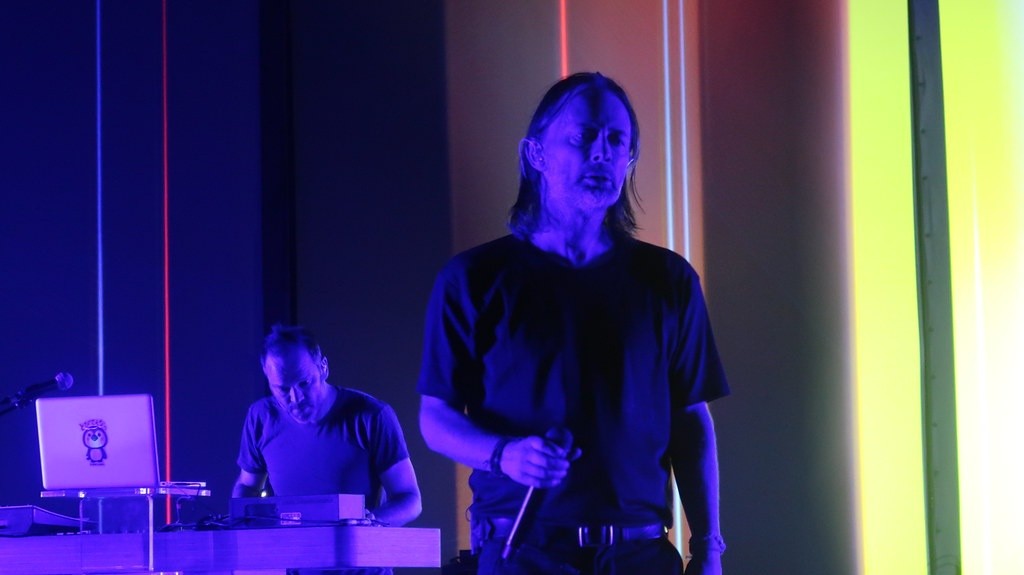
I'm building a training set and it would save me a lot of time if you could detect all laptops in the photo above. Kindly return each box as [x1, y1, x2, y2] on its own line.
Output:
[35, 393, 207, 488]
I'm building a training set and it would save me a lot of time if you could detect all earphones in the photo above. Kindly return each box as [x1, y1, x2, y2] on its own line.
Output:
[534, 151, 544, 163]
[322, 365, 327, 374]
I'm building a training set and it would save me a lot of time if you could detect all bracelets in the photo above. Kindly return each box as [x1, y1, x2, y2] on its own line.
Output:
[689, 528, 726, 555]
[490, 436, 513, 479]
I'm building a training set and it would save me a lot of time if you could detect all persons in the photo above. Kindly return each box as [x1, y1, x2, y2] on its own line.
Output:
[416, 73, 730, 575]
[232, 326, 422, 575]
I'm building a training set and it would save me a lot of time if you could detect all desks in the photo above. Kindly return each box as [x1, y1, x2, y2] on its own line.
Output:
[0, 525, 441, 575]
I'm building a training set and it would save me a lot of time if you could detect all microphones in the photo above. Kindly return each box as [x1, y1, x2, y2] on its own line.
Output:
[17, 371, 73, 400]
[502, 428, 571, 561]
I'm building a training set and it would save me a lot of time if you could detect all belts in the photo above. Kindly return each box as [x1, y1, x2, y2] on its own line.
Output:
[475, 516, 668, 551]
